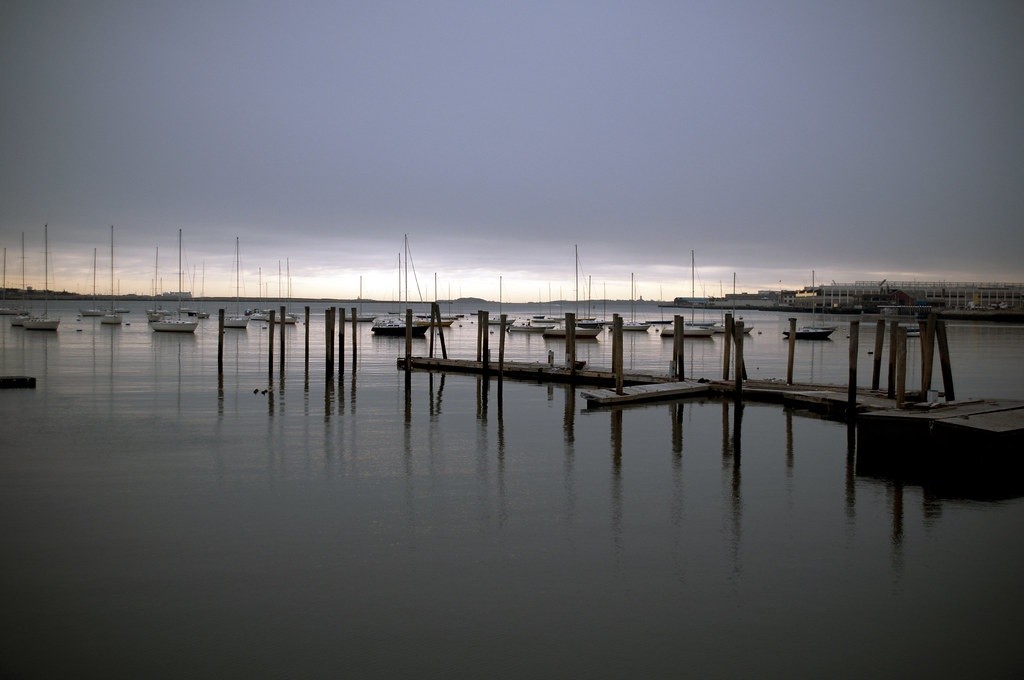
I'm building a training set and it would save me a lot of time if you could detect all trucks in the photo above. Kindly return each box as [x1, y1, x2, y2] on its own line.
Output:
[965, 302, 994, 311]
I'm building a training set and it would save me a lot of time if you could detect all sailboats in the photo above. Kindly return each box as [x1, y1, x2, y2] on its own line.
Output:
[0, 222, 924, 341]
